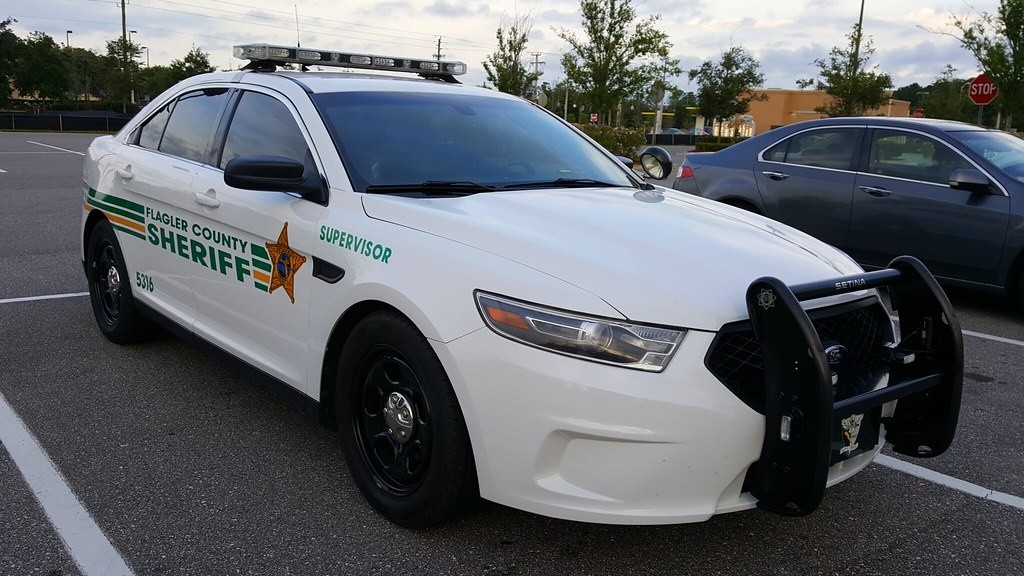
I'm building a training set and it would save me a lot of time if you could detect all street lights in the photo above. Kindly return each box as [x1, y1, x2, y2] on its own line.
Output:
[565, 79, 572, 120]
[129, 30, 138, 102]
[67, 30, 72, 47]
[141, 47, 149, 67]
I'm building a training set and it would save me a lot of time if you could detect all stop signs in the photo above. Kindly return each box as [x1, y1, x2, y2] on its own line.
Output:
[914, 109, 925, 118]
[968, 75, 998, 106]
[590, 113, 597, 122]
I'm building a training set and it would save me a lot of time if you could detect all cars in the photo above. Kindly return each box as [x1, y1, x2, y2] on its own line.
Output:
[661, 126, 712, 135]
[672, 116, 1024, 305]
[82, 42, 964, 533]
